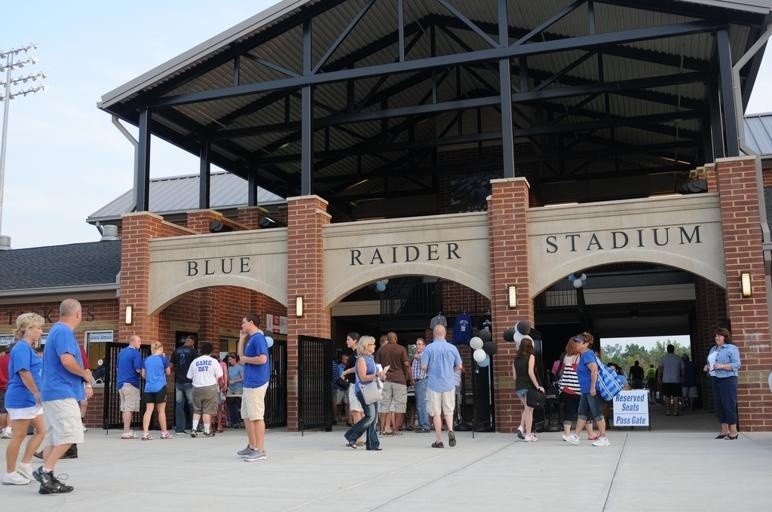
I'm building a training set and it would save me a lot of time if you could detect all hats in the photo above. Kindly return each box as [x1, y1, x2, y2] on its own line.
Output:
[572, 334, 586, 343]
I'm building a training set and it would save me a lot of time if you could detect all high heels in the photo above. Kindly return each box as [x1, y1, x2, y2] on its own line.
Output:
[715, 434, 726, 439]
[725, 434, 738, 440]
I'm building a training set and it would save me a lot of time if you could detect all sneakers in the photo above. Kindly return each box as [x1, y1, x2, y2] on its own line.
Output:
[236, 444, 267, 462]
[16, 463, 37, 481]
[59, 444, 77, 459]
[333, 418, 350, 426]
[561, 433, 610, 446]
[2, 471, 31, 485]
[517, 425, 525, 439]
[346, 440, 382, 450]
[379, 424, 443, 436]
[82, 423, 88, 432]
[663, 409, 679, 416]
[121, 427, 223, 439]
[0, 431, 14, 439]
[524, 432, 538, 442]
[448, 431, 456, 447]
[32, 465, 73, 494]
[432, 440, 443, 448]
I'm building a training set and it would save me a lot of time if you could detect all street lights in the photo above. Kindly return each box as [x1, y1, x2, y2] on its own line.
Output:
[0, 35, 49, 239]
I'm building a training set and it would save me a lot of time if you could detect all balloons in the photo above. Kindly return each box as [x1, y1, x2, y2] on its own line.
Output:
[470, 330, 497, 367]
[376, 282, 386, 292]
[381, 279, 389, 285]
[503, 321, 536, 347]
[568, 274, 577, 282]
[573, 279, 582, 288]
[574, 271, 582, 279]
[580, 273, 586, 280]
[376, 280, 381, 284]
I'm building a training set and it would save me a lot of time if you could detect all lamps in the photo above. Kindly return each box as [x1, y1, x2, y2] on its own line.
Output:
[508, 284, 517, 308]
[125, 304, 133, 326]
[295, 295, 304, 318]
[740, 273, 752, 298]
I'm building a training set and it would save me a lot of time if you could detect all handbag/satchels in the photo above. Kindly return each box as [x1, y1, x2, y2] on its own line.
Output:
[359, 380, 383, 405]
[336, 376, 349, 391]
[526, 388, 545, 409]
[596, 364, 626, 403]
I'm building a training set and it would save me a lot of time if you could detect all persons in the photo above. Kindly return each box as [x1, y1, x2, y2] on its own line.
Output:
[1, 311, 50, 486]
[513, 339, 544, 442]
[629, 345, 701, 415]
[30, 297, 96, 495]
[332, 324, 462, 450]
[552, 332, 610, 445]
[702, 325, 745, 441]
[116, 336, 244, 440]
[0, 344, 94, 457]
[234, 311, 273, 465]
